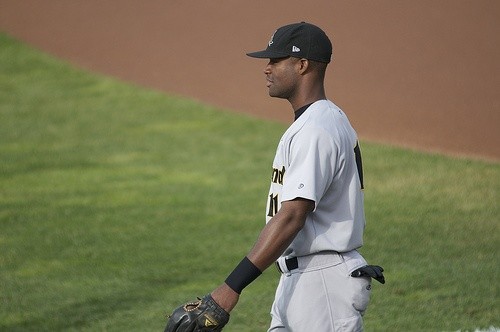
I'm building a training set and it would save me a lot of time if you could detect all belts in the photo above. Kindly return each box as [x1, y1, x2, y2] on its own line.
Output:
[277, 257, 298, 274]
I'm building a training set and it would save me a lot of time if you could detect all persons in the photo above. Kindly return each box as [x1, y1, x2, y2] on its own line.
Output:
[165, 20, 386, 331]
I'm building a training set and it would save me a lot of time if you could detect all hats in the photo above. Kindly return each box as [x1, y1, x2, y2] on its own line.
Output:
[246, 21, 332, 63]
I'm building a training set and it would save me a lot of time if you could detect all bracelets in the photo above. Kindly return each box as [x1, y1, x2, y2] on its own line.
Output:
[225, 256, 263, 296]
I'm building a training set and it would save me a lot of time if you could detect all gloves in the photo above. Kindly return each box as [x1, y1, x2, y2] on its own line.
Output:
[351, 265, 385, 284]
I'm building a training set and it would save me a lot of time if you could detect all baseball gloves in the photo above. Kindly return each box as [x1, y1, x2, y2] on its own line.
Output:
[164, 293, 230, 332]
